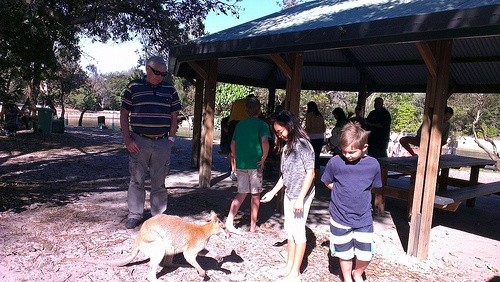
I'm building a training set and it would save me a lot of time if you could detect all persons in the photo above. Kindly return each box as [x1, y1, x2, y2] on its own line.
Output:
[260, 110, 316, 282]
[441, 137, 456, 155]
[347, 105, 366, 130]
[0, 98, 23, 148]
[304, 101, 326, 185]
[449, 125, 458, 148]
[365, 97, 391, 184]
[120, 56, 182, 229]
[225, 95, 272, 235]
[326, 107, 346, 157]
[267, 100, 286, 180]
[221, 116, 237, 181]
[225, 94, 255, 173]
[321, 121, 382, 282]
[399, 106, 457, 156]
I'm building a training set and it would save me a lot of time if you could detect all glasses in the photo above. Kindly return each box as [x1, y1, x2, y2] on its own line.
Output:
[276, 128, 285, 136]
[148, 65, 168, 77]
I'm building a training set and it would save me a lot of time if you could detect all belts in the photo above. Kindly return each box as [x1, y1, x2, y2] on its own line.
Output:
[133, 130, 168, 141]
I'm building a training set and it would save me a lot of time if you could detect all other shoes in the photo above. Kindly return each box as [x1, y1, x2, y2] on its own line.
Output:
[229, 173, 237, 181]
[125, 219, 139, 229]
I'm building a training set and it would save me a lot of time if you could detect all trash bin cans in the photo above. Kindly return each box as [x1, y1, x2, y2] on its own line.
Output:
[36, 107, 54, 136]
[33, 118, 65, 133]
[98, 116, 105, 126]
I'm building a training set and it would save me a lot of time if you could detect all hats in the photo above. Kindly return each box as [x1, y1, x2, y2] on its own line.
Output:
[374, 98, 383, 102]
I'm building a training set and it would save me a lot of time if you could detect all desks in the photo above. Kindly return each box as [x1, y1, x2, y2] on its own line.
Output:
[378, 154, 497, 220]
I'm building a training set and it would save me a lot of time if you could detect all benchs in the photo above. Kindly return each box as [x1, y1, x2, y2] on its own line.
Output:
[371, 186, 462, 212]
[437, 181, 500, 208]
